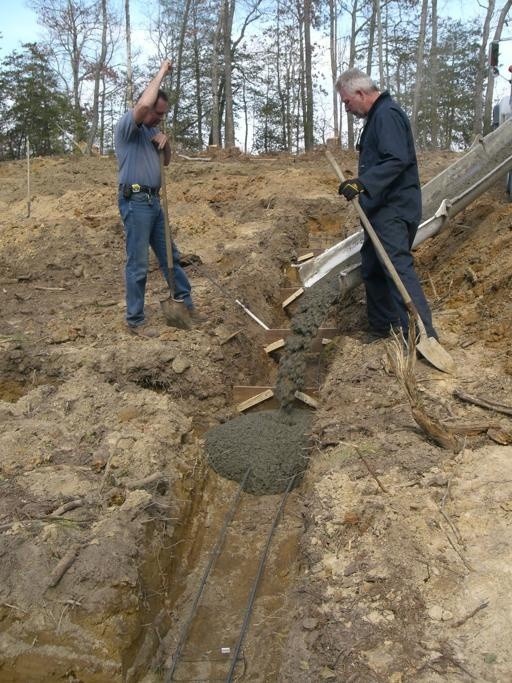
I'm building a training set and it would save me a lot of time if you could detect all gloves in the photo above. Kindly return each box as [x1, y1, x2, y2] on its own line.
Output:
[338, 179, 364, 201]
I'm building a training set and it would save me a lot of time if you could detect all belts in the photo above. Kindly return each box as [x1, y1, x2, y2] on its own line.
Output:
[118, 184, 159, 194]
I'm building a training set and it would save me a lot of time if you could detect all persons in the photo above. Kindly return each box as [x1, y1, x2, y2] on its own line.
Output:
[113, 58, 209, 340]
[335, 67, 439, 360]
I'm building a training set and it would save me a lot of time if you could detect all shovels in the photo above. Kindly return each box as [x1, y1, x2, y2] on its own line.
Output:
[325, 150, 455, 374]
[158, 147, 191, 331]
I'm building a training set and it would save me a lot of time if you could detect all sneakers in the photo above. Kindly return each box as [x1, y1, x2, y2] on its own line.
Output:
[131, 324, 159, 340]
[188, 308, 208, 321]
